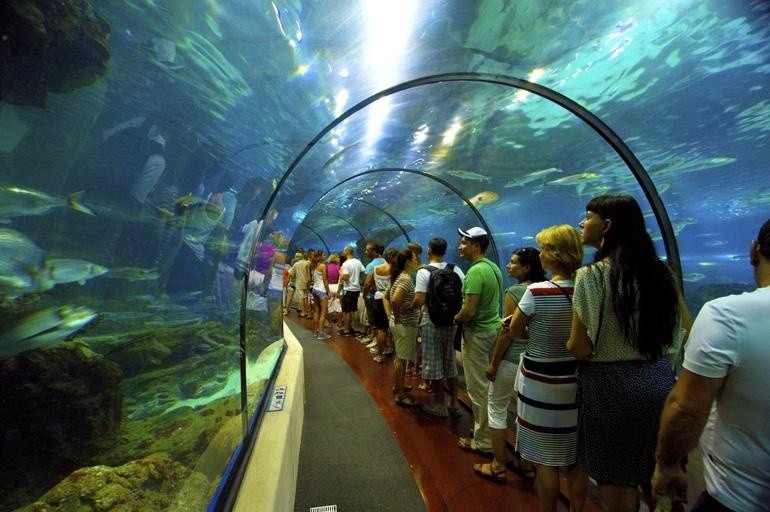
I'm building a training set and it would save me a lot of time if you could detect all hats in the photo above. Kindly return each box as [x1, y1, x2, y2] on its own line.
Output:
[293, 253, 302, 262]
[458, 227, 488, 239]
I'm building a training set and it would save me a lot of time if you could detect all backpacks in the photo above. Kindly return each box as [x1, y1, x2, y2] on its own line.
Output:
[416, 263, 463, 328]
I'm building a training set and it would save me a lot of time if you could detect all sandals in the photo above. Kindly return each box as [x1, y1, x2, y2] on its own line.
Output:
[395, 394, 419, 409]
[457, 437, 494, 460]
[507, 458, 535, 480]
[473, 463, 506, 484]
[392, 385, 412, 393]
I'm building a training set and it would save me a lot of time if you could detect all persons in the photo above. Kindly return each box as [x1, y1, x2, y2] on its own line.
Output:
[312, 250, 332, 339]
[284, 248, 316, 319]
[471, 248, 547, 484]
[406, 244, 423, 378]
[651, 219, 770, 512]
[383, 250, 419, 407]
[566, 195, 693, 512]
[453, 227, 503, 457]
[501, 224, 588, 512]
[355, 244, 384, 344]
[337, 246, 365, 337]
[402, 237, 466, 418]
[326, 253, 343, 328]
[373, 247, 401, 363]
[363, 243, 388, 352]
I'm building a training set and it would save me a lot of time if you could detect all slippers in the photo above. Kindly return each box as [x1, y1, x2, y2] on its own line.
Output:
[308, 317, 313, 319]
[297, 313, 305, 317]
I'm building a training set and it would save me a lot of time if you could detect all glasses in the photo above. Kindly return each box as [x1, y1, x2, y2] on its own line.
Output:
[518, 247, 532, 258]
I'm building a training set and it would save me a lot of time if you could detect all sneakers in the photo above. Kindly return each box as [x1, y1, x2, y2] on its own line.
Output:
[369, 347, 382, 353]
[314, 332, 318, 336]
[336, 329, 350, 336]
[447, 402, 463, 416]
[422, 404, 449, 417]
[336, 323, 345, 330]
[382, 346, 393, 355]
[317, 333, 331, 339]
[418, 383, 433, 393]
[373, 357, 383, 363]
[353, 331, 361, 340]
[283, 308, 286, 312]
[406, 371, 411, 377]
[360, 337, 374, 345]
[412, 371, 419, 379]
[328, 324, 331, 327]
[282, 311, 288, 315]
[366, 340, 378, 349]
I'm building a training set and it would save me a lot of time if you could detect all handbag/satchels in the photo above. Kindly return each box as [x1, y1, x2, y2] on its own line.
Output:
[453, 260, 501, 352]
[548, 279, 573, 307]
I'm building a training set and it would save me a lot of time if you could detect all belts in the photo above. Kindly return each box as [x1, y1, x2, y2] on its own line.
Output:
[378, 289, 386, 291]
